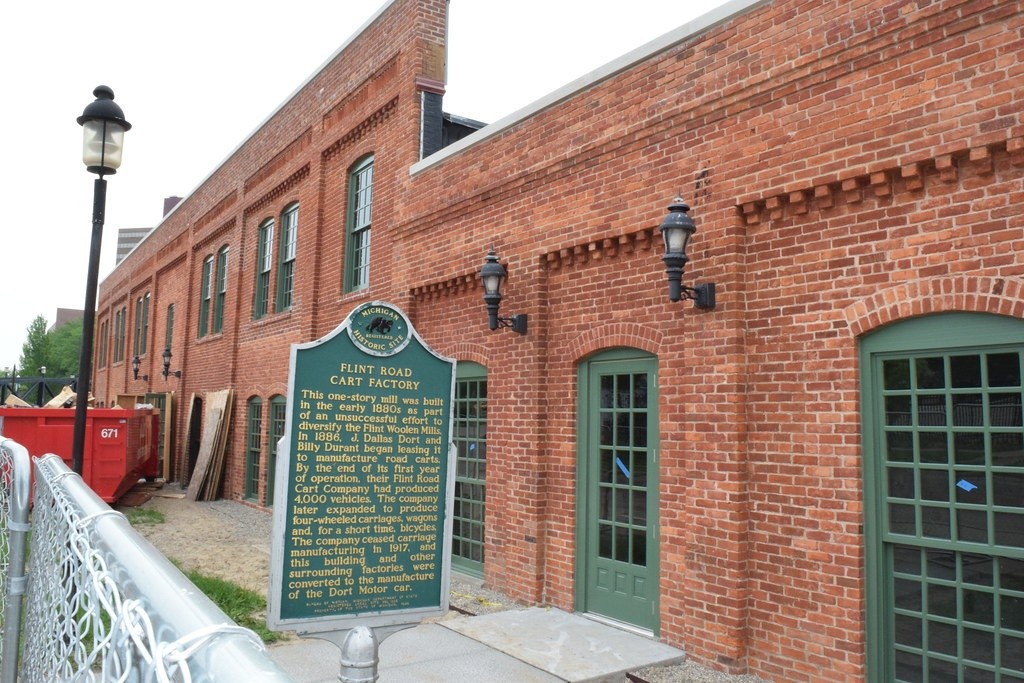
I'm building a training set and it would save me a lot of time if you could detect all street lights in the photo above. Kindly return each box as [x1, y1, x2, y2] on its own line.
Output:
[53, 85, 132, 655]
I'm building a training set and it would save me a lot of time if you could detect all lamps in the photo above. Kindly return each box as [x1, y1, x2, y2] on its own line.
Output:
[132, 356, 148, 381]
[478, 245, 528, 333]
[660, 187, 716, 309]
[162, 345, 181, 378]
